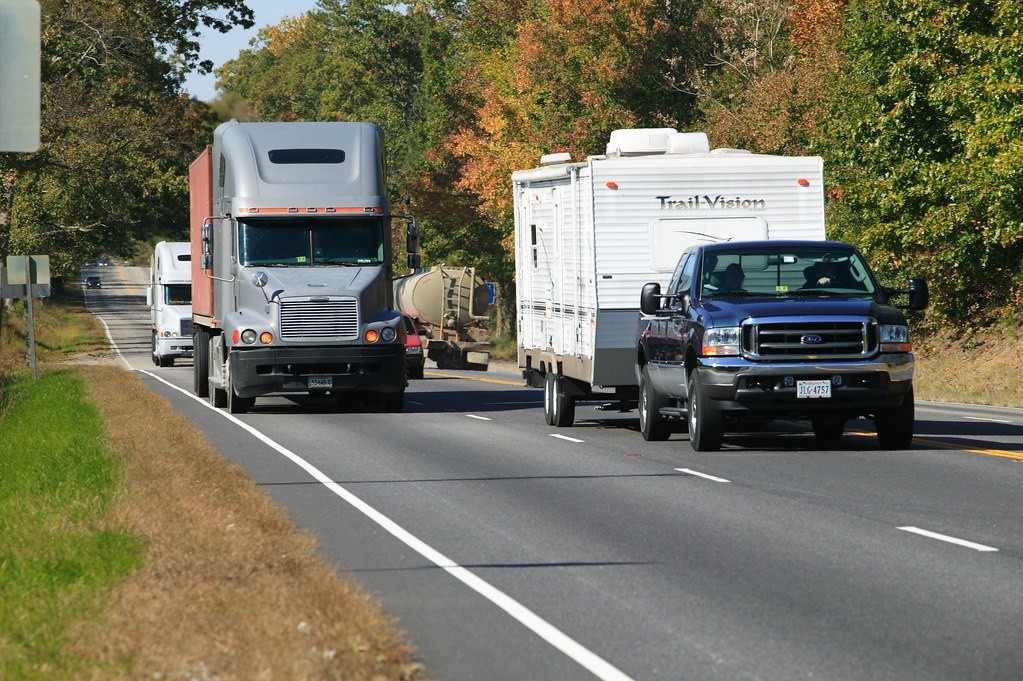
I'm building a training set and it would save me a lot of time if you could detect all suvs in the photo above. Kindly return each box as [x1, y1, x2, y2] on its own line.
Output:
[632, 237, 930, 452]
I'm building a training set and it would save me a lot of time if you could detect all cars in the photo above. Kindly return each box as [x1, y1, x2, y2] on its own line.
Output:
[85, 276, 102, 289]
[84, 255, 134, 267]
[397, 311, 428, 379]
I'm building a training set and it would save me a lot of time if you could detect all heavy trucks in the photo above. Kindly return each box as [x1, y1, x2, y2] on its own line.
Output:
[186, 119, 421, 414]
[148, 240, 197, 368]
[391, 264, 492, 371]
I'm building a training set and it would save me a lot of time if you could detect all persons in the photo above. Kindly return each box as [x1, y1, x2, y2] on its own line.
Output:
[717, 263, 748, 292]
[802, 261, 846, 288]
